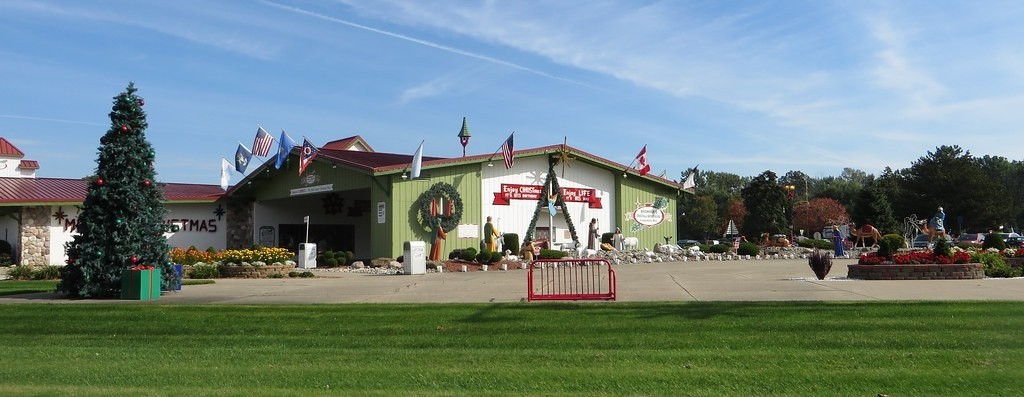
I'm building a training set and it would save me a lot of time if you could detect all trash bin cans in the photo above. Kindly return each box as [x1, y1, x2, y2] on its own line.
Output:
[403, 240, 426, 275]
[298, 242, 317, 268]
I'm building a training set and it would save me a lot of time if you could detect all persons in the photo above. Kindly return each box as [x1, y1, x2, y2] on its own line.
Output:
[588, 218, 600, 249]
[429, 218, 446, 261]
[524, 238, 534, 260]
[930, 206, 946, 230]
[610, 227, 625, 251]
[484, 216, 500, 252]
[831, 225, 844, 257]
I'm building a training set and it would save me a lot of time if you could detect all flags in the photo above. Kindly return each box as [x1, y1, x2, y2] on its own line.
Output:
[251, 127, 274, 157]
[411, 144, 423, 180]
[502, 135, 514, 169]
[658, 170, 666, 179]
[635, 146, 650, 176]
[299, 138, 319, 175]
[235, 144, 252, 173]
[221, 158, 236, 191]
[684, 168, 696, 190]
[275, 130, 295, 169]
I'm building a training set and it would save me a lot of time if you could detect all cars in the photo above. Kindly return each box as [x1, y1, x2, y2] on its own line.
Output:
[677, 240, 701, 248]
[953, 233, 985, 245]
[999, 232, 1021, 243]
[910, 234, 955, 249]
